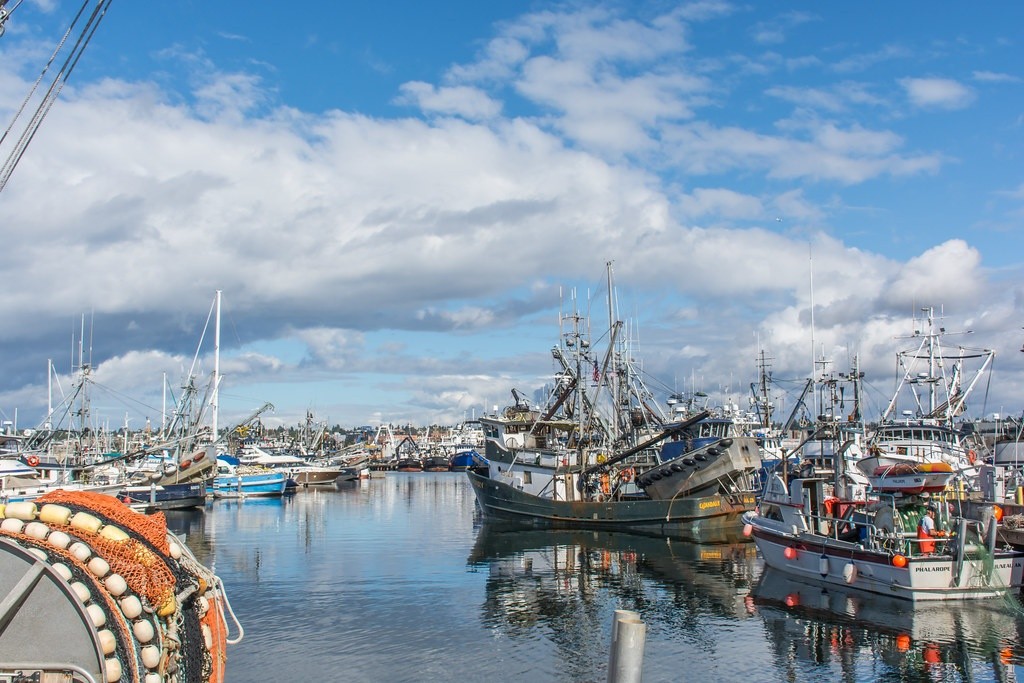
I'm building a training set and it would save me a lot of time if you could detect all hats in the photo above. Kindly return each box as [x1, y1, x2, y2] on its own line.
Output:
[927, 506, 938, 512]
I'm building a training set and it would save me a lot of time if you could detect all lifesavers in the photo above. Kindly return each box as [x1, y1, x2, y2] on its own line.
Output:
[971, 450, 976, 461]
[621, 469, 631, 482]
[27, 455, 39, 466]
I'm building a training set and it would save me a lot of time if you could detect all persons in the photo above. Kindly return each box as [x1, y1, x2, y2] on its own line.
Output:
[918, 506, 946, 552]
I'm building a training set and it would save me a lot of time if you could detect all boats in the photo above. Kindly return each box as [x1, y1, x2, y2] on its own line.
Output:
[0, 242, 1024, 603]
[470, 522, 741, 628]
[747, 562, 1024, 683]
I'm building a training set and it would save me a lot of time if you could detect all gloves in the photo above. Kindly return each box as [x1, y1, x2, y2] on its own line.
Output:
[938, 530, 946, 536]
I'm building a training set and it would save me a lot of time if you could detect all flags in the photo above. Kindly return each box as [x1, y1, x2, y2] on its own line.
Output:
[593, 356, 600, 382]
